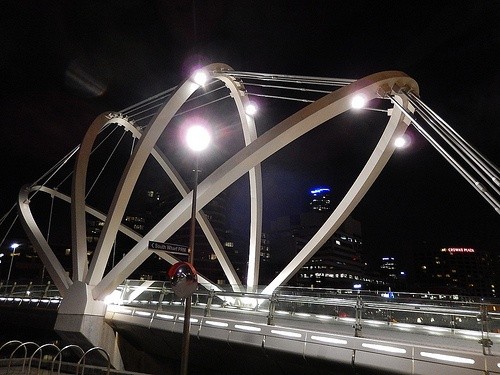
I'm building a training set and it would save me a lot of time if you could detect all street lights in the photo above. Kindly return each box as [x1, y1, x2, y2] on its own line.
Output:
[4, 243, 19, 294]
[180, 124, 210, 374]
